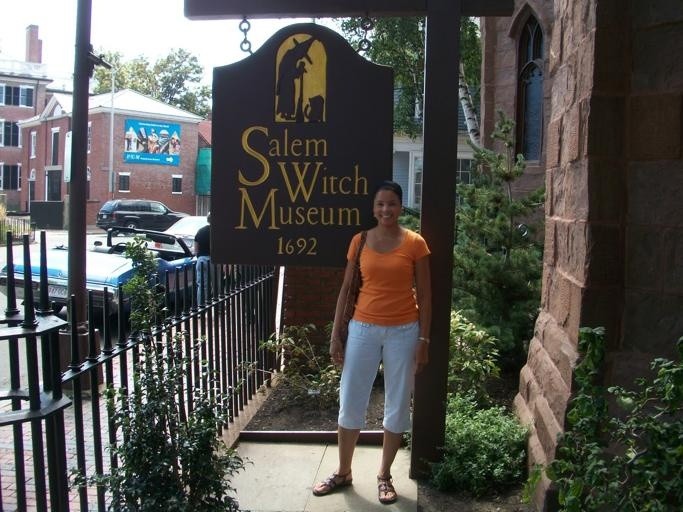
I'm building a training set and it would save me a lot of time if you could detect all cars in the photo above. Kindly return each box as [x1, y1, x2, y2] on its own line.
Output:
[97, 198, 210, 253]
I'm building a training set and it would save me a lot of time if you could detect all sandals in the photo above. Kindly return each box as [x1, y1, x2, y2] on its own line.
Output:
[376, 473, 397, 503]
[311, 468, 352, 496]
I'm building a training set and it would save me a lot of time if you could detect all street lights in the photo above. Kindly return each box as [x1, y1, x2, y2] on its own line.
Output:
[108, 53, 142, 198]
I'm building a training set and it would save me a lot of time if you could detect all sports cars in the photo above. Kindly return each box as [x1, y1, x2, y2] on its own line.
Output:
[0, 226, 197, 314]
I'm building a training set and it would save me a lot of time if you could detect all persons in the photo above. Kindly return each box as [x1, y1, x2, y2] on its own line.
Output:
[313, 181, 432, 502]
[194, 212, 210, 306]
[277, 45, 304, 120]
[125, 126, 180, 154]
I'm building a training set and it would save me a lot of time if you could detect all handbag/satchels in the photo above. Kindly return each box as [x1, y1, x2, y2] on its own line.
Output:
[341, 228, 369, 325]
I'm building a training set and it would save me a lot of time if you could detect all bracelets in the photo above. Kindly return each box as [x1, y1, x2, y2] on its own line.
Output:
[416, 337, 430, 344]
[329, 337, 341, 343]
